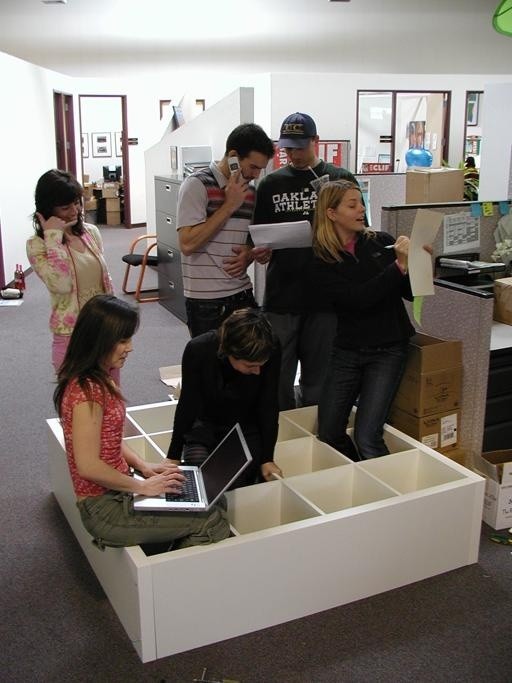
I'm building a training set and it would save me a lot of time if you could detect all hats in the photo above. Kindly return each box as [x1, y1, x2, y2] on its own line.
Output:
[278, 111, 316, 149]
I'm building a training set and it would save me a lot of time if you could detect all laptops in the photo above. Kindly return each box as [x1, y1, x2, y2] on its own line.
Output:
[132, 422, 253, 512]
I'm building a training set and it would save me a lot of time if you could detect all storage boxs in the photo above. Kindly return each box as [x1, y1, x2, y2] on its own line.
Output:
[389, 405, 462, 454]
[106, 199, 120, 212]
[102, 183, 118, 198]
[475, 448, 512, 530]
[389, 330, 464, 419]
[107, 212, 120, 225]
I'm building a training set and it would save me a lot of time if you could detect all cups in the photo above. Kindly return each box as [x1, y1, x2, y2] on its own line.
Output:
[311, 174, 329, 191]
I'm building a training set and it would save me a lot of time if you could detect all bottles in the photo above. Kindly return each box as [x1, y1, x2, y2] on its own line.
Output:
[19, 265, 25, 289]
[14, 264, 19, 289]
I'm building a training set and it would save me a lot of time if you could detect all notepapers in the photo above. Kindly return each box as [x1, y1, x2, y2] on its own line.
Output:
[483, 202, 495, 217]
[470, 204, 482, 217]
[499, 201, 509, 216]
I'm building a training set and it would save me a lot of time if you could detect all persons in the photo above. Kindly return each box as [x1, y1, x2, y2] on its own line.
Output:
[313, 181, 433, 463]
[176, 122, 275, 341]
[166, 308, 285, 490]
[26, 167, 115, 396]
[246, 110, 370, 411]
[53, 295, 230, 550]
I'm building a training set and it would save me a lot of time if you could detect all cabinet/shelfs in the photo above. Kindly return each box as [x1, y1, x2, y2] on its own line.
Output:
[44, 400, 486, 664]
[154, 175, 189, 325]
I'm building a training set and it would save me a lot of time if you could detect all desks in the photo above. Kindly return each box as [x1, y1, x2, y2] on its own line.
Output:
[380, 200, 512, 471]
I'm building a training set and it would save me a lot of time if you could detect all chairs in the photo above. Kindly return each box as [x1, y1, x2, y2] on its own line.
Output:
[122, 233, 159, 303]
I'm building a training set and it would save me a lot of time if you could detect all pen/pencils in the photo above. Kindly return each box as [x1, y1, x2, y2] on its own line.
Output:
[385, 243, 395, 249]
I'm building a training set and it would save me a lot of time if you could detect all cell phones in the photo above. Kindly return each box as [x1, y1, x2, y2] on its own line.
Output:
[225, 156, 244, 184]
